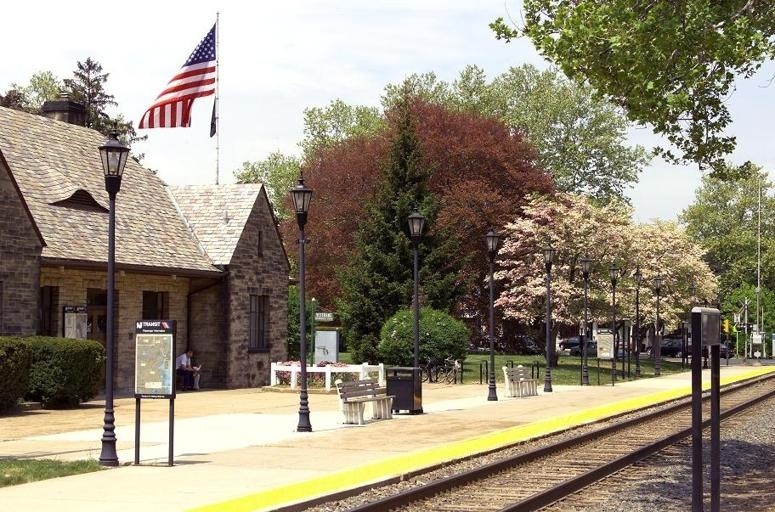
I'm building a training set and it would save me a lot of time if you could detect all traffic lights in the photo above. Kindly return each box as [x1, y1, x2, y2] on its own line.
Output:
[722, 320, 728, 333]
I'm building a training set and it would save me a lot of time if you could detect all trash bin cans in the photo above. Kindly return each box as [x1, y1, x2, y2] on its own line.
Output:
[386, 367, 423, 415]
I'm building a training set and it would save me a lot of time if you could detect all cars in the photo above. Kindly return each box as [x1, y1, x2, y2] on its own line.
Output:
[645, 338, 735, 358]
[558, 336, 626, 360]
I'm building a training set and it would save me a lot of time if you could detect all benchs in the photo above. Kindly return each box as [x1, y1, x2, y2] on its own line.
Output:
[336, 377, 397, 425]
[501, 364, 539, 399]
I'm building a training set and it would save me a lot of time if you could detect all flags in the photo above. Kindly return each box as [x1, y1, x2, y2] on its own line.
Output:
[138, 23, 216, 129]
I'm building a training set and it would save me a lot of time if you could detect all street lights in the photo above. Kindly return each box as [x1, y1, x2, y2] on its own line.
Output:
[406, 205, 426, 411]
[485, 227, 498, 403]
[96, 137, 129, 464]
[289, 169, 314, 430]
[542, 242, 557, 393]
[580, 252, 686, 386]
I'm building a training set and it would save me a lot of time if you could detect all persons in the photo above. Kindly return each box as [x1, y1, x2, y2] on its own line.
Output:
[176, 349, 202, 390]
